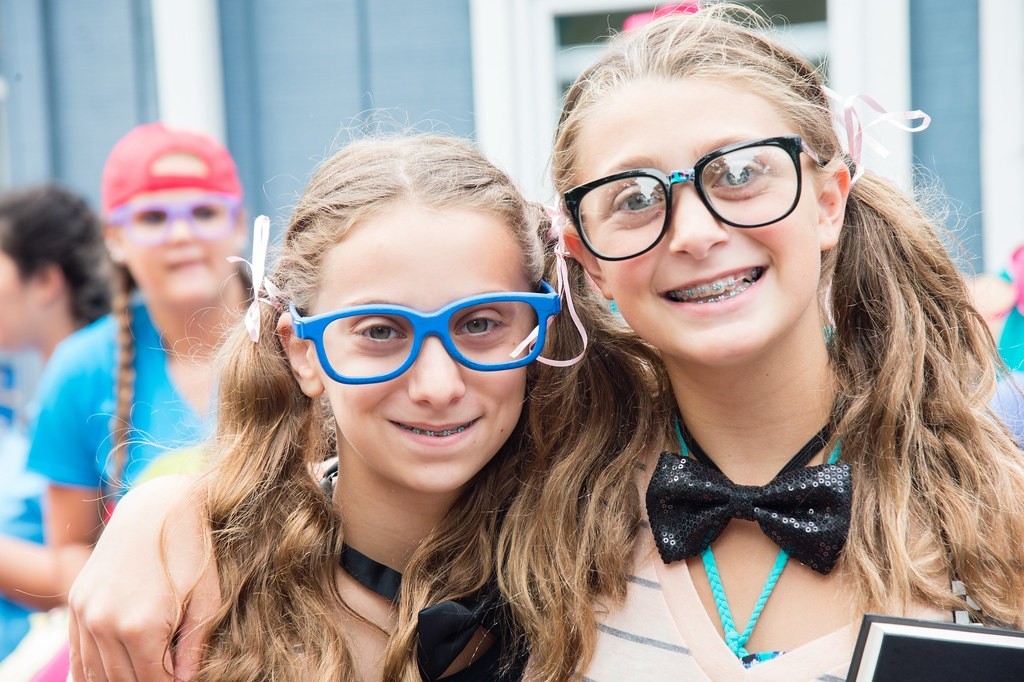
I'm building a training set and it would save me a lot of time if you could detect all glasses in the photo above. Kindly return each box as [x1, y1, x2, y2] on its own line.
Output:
[284, 280, 565, 384]
[558, 134, 836, 262]
[101, 199, 247, 239]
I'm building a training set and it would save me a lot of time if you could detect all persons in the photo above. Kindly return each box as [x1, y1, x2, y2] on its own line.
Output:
[24, 122, 274, 552]
[67, 0, 1022, 682]
[158, 107, 679, 682]
[0, 186, 113, 667]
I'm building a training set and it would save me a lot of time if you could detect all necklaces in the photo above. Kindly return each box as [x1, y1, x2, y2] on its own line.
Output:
[673, 324, 845, 669]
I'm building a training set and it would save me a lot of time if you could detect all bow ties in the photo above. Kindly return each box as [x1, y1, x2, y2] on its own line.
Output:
[643, 447, 856, 576]
[409, 576, 509, 681]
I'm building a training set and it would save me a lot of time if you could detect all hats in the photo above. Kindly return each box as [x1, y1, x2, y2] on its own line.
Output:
[101, 121, 245, 214]
